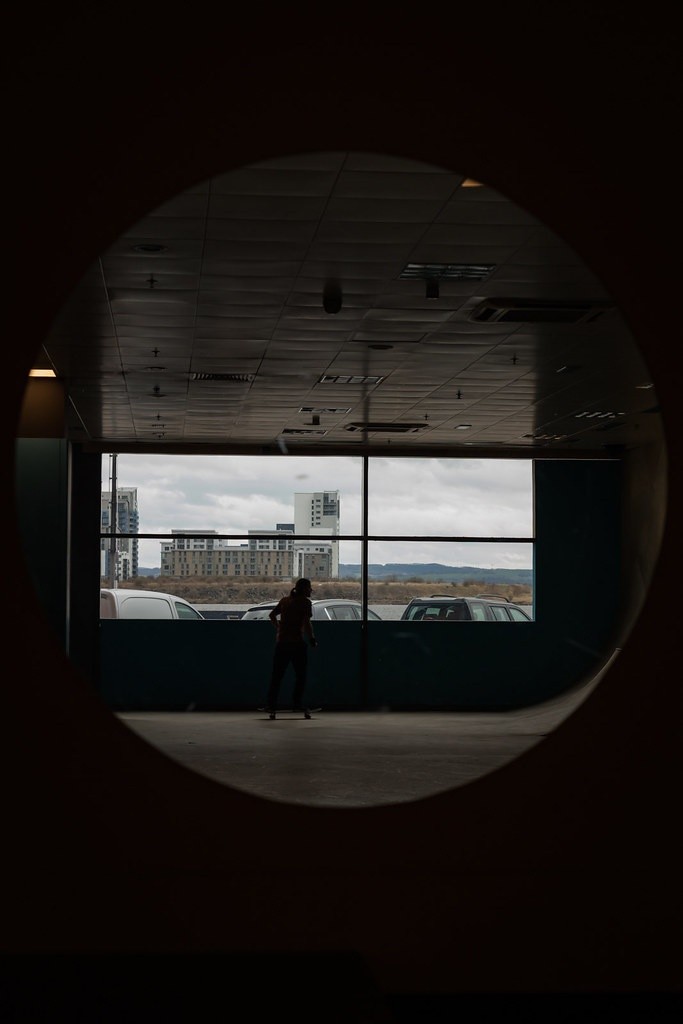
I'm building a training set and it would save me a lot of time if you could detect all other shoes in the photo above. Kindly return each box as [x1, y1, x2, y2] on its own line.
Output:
[293, 698, 303, 707]
[267, 699, 279, 706]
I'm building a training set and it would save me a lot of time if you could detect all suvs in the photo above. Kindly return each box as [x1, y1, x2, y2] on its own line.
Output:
[400, 593, 533, 621]
[241, 599, 383, 620]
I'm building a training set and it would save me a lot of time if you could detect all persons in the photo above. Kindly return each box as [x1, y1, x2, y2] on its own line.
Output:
[266, 578, 315, 710]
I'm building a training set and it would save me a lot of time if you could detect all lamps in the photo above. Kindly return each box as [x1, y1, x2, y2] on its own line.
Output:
[323, 297, 342, 314]
[311, 416, 321, 425]
[425, 280, 440, 301]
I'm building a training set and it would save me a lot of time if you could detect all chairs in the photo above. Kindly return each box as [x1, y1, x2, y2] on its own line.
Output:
[446, 608, 465, 619]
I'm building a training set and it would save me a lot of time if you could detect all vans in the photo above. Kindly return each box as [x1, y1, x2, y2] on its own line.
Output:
[100, 581, 205, 620]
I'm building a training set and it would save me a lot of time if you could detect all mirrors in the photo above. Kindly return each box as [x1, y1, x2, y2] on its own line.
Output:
[9, 150, 668, 810]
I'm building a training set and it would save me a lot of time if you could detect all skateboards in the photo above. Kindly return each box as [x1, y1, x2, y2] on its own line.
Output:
[259, 708, 324, 720]
